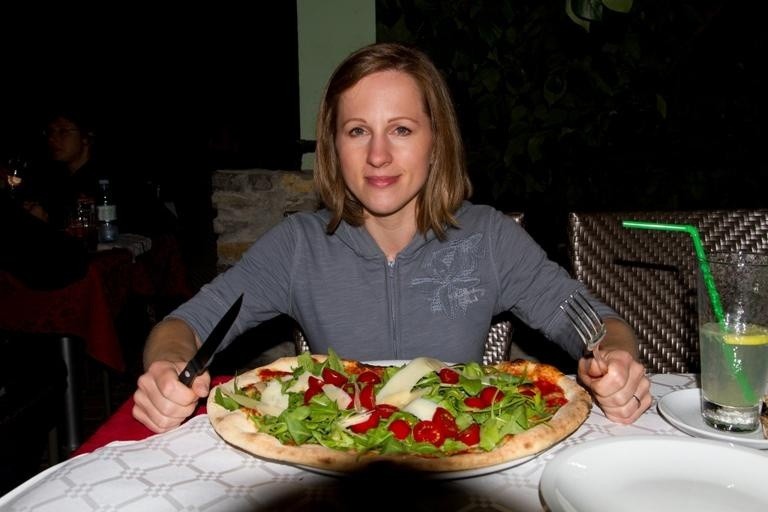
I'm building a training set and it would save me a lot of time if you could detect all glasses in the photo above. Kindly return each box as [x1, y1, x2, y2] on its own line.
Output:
[47, 127, 82, 136]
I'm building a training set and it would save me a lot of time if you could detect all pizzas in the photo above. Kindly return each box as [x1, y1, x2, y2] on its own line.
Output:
[206, 354, 594, 473]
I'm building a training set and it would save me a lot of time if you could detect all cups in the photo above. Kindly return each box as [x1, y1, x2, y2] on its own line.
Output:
[694, 252, 766, 434]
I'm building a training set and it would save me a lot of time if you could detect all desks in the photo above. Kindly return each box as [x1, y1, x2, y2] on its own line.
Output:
[0, 234, 154, 452]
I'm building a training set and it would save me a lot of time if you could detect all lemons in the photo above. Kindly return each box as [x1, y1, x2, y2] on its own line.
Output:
[699, 322, 768, 345]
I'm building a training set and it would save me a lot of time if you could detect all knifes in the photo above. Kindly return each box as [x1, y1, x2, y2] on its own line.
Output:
[180, 291, 245, 385]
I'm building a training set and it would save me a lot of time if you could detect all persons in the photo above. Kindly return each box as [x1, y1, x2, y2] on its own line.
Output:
[1, 190, 70, 293]
[28, 106, 182, 234]
[130, 43, 656, 435]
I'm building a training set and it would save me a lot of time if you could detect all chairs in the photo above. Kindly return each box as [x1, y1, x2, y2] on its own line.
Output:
[569, 209, 766, 375]
[298, 208, 526, 373]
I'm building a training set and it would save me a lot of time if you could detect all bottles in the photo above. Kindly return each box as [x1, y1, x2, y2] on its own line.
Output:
[97, 180, 120, 242]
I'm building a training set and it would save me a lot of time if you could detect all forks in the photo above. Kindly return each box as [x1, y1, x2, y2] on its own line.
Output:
[559, 288, 608, 373]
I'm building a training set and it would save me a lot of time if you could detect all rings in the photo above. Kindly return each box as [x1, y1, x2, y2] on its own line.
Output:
[632, 395, 641, 408]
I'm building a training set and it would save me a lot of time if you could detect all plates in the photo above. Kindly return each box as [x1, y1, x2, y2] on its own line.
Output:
[539, 433, 768, 512]
[657, 387, 768, 450]
[294, 357, 552, 481]
